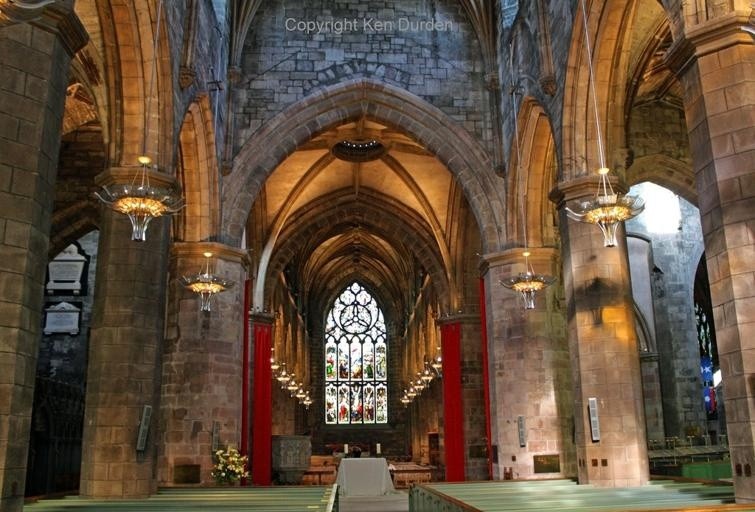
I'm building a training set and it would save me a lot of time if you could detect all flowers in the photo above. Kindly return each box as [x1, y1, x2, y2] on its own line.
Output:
[209, 446, 250, 486]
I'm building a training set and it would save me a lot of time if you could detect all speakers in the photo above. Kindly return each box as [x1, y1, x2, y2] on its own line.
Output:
[137, 405, 152, 451]
[212, 420, 221, 452]
[588, 397, 600, 440]
[518, 416, 526, 447]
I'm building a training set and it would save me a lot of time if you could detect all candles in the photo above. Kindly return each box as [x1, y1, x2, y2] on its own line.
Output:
[376, 443, 381, 454]
[343, 443, 349, 454]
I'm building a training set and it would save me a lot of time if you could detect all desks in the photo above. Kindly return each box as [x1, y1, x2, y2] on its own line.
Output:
[335, 457, 394, 496]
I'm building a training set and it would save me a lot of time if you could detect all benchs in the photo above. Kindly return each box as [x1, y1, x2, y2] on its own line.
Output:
[0, 483, 336, 512]
[387, 459, 432, 489]
[303, 455, 338, 485]
[408, 478, 755, 512]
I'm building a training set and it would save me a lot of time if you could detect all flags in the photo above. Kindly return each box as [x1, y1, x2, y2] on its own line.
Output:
[700, 357, 713, 381]
[703, 387, 715, 411]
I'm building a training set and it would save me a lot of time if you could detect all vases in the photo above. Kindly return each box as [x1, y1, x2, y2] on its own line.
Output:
[222, 481, 235, 488]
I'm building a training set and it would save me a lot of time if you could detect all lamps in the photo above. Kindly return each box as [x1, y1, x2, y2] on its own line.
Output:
[561, 0, 646, 249]
[497, 41, 558, 311]
[270, 348, 313, 410]
[91, 0, 190, 243]
[175, 36, 237, 313]
[399, 345, 443, 409]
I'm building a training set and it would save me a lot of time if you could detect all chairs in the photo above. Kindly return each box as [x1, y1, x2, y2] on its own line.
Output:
[648, 434, 729, 468]
